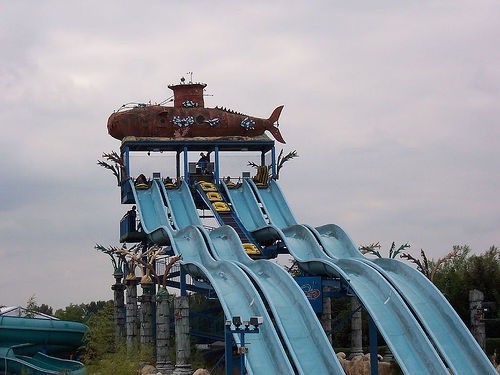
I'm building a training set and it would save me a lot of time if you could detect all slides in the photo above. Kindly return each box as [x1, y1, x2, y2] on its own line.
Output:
[0, 347, 87, 375]
[129, 178, 500, 375]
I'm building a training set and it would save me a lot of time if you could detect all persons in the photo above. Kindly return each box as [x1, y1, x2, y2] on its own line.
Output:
[136, 174, 149, 185]
[164, 177, 174, 184]
[225, 176, 235, 185]
[141, 365, 157, 375]
[127, 206, 137, 230]
[193, 368, 210, 375]
[196, 150, 213, 170]
